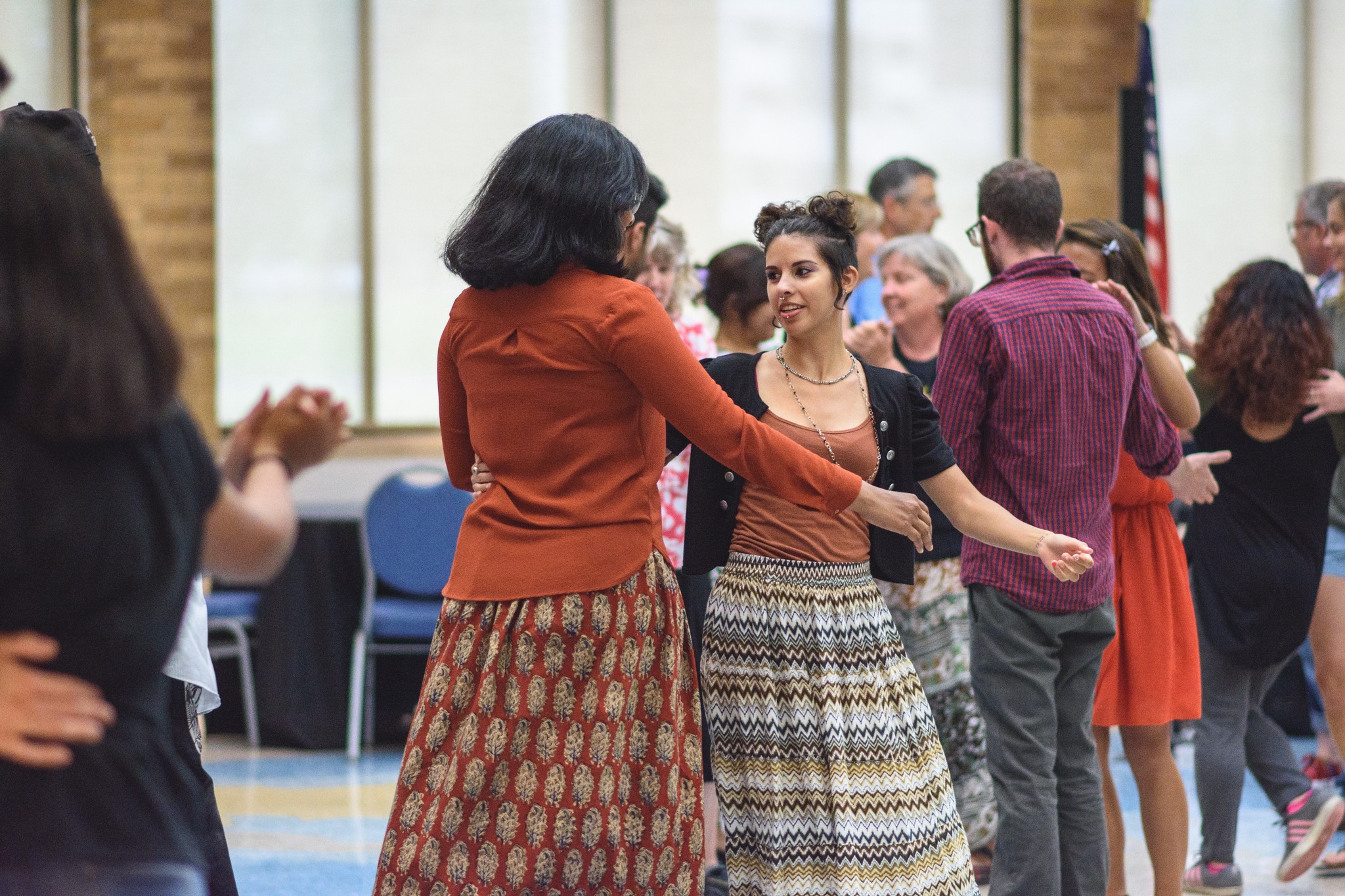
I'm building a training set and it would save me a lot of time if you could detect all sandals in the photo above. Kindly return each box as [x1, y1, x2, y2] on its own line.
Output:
[1313, 848, 1345, 877]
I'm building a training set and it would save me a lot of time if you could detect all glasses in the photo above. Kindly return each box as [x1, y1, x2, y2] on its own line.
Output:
[966, 215, 993, 247]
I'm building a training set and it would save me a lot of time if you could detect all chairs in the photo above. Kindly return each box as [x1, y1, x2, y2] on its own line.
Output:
[342, 464, 475, 769]
[205, 590, 266, 747]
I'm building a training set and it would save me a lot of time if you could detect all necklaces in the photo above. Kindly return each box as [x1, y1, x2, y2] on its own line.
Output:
[780, 344, 881, 485]
[774, 344, 855, 384]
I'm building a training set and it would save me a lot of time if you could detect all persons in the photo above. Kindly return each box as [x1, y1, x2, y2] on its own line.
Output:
[0, 50, 1345, 896]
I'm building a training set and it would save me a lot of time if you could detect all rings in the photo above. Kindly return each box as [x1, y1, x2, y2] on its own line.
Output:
[472, 465, 478, 475]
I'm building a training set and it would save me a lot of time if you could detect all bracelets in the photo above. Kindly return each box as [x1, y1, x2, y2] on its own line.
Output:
[250, 450, 293, 478]
[1135, 324, 1158, 348]
[1035, 532, 1052, 552]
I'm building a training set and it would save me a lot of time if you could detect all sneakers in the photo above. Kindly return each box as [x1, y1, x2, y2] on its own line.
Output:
[1183, 860, 1243, 896]
[1299, 754, 1345, 789]
[1271, 789, 1345, 880]
